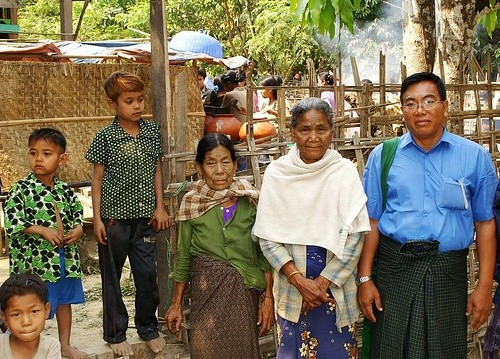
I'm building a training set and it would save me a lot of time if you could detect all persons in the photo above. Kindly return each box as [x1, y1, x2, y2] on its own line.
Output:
[85, 71, 172, 356]
[5, 128, 89, 359]
[164, 133, 276, 359]
[357, 72, 499, 359]
[0, 274, 62, 359]
[251, 97, 371, 359]
[197, 68, 292, 132]
[317, 72, 335, 138]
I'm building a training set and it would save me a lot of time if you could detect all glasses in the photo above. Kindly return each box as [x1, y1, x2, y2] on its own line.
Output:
[401, 98, 443, 113]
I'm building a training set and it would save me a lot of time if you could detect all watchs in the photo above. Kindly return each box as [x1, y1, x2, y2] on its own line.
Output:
[355, 275, 372, 286]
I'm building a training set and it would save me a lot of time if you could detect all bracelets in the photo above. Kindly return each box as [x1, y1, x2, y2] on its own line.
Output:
[264, 292, 274, 300]
[287, 271, 303, 284]
